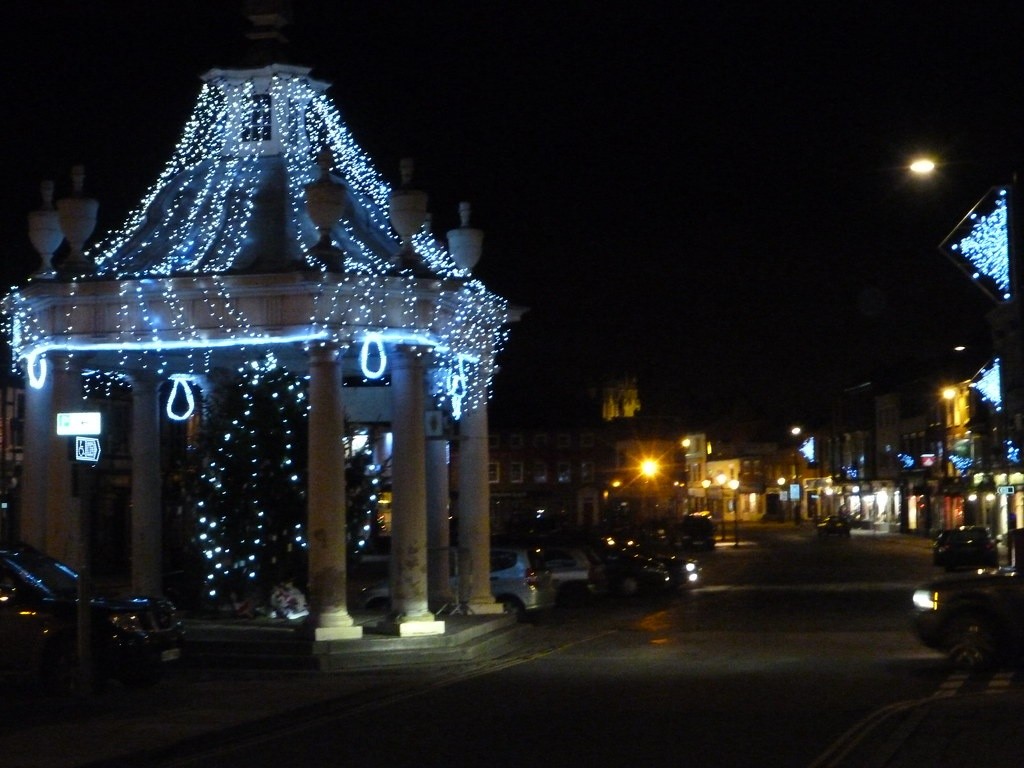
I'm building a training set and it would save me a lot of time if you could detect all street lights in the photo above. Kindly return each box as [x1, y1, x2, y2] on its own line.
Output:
[942, 384, 957, 528]
[791, 426, 803, 526]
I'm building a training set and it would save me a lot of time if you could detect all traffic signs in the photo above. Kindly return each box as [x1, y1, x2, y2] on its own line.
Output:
[75, 437, 102, 463]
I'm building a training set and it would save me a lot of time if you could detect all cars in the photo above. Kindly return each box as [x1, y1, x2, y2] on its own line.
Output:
[446, 514, 717, 623]
[930, 525, 1003, 574]
[815, 514, 852, 537]
[905, 565, 1024, 672]
[0, 537, 186, 676]
